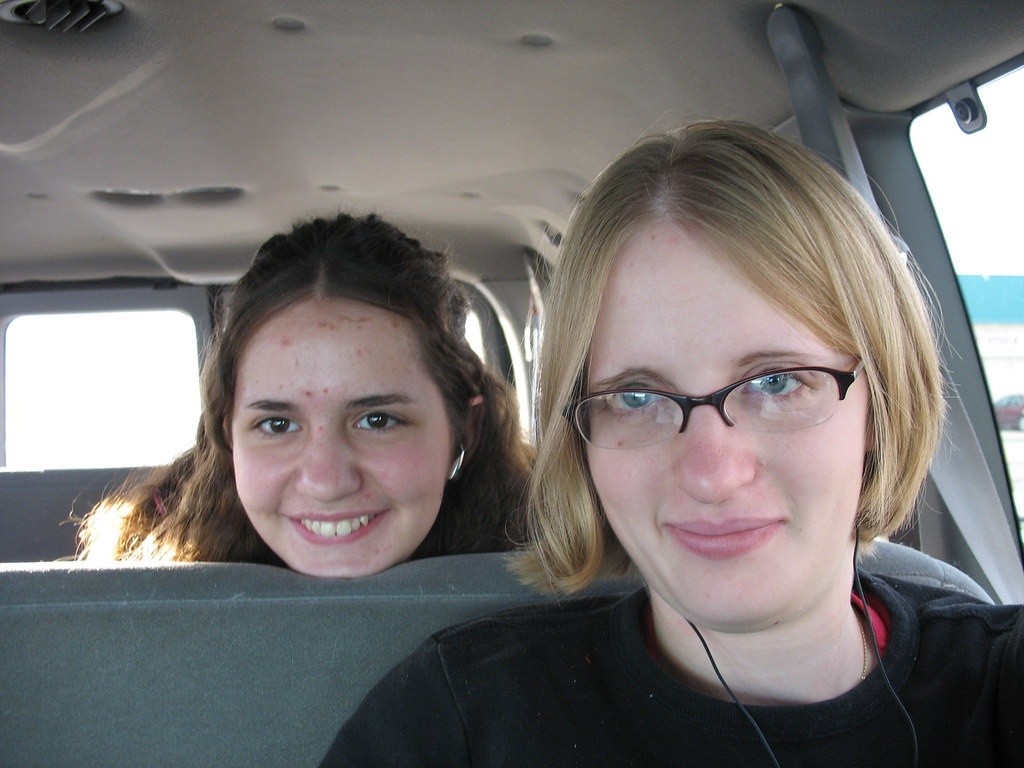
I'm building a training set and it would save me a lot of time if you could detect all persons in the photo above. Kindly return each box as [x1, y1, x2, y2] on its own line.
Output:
[317, 119, 1023, 768]
[65, 211, 534, 579]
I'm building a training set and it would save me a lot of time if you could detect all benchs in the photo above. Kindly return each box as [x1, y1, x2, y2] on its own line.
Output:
[0, 540, 995, 768]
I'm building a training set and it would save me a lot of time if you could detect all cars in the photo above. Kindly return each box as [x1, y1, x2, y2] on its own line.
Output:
[993, 394, 1024, 431]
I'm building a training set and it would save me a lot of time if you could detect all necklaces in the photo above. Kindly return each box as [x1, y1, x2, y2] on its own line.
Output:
[851, 603, 867, 687]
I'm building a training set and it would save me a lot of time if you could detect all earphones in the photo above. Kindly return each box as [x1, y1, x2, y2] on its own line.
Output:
[447, 437, 465, 479]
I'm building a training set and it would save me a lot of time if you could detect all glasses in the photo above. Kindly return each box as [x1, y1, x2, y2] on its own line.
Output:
[561, 358, 864, 448]
